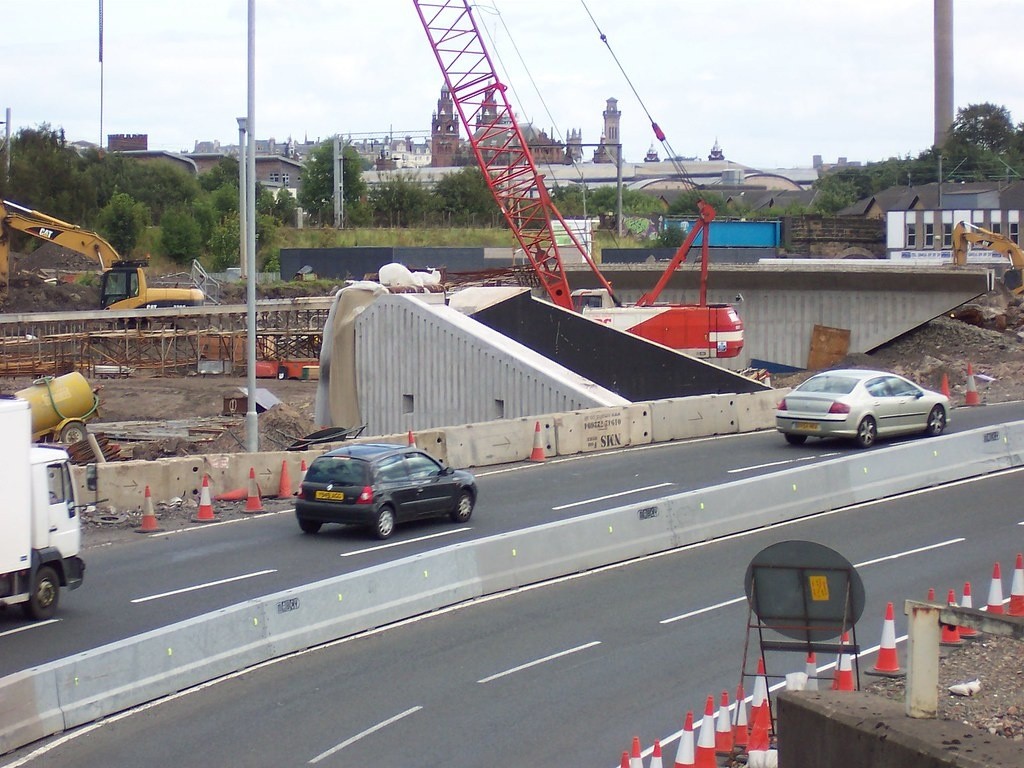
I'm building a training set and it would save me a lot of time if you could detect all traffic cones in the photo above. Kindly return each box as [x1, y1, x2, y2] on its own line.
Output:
[714, 689, 735, 757]
[673, 711, 695, 768]
[863, 601, 908, 678]
[1007, 551, 1024, 617]
[620, 751, 630, 768]
[649, 738, 663, 768]
[526, 422, 549, 462]
[693, 695, 718, 768]
[629, 736, 645, 768]
[409, 430, 418, 448]
[731, 683, 750, 748]
[190, 474, 221, 523]
[271, 460, 296, 500]
[957, 582, 983, 639]
[240, 466, 269, 514]
[830, 631, 855, 692]
[986, 562, 1006, 614]
[736, 697, 768, 764]
[926, 587, 936, 604]
[212, 481, 263, 501]
[958, 361, 986, 407]
[296, 459, 309, 498]
[939, 589, 969, 648]
[747, 656, 773, 737]
[806, 652, 818, 692]
[134, 485, 167, 534]
[941, 372, 950, 401]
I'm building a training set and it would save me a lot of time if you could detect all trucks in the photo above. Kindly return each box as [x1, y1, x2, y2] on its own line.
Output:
[0, 392, 98, 620]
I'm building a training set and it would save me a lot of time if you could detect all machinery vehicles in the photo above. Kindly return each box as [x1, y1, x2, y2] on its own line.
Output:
[411, 0, 753, 375]
[941, 221, 1024, 302]
[0, 199, 204, 328]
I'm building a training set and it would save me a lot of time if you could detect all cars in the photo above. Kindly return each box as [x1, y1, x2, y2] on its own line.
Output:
[295, 442, 478, 540]
[774, 369, 950, 449]
